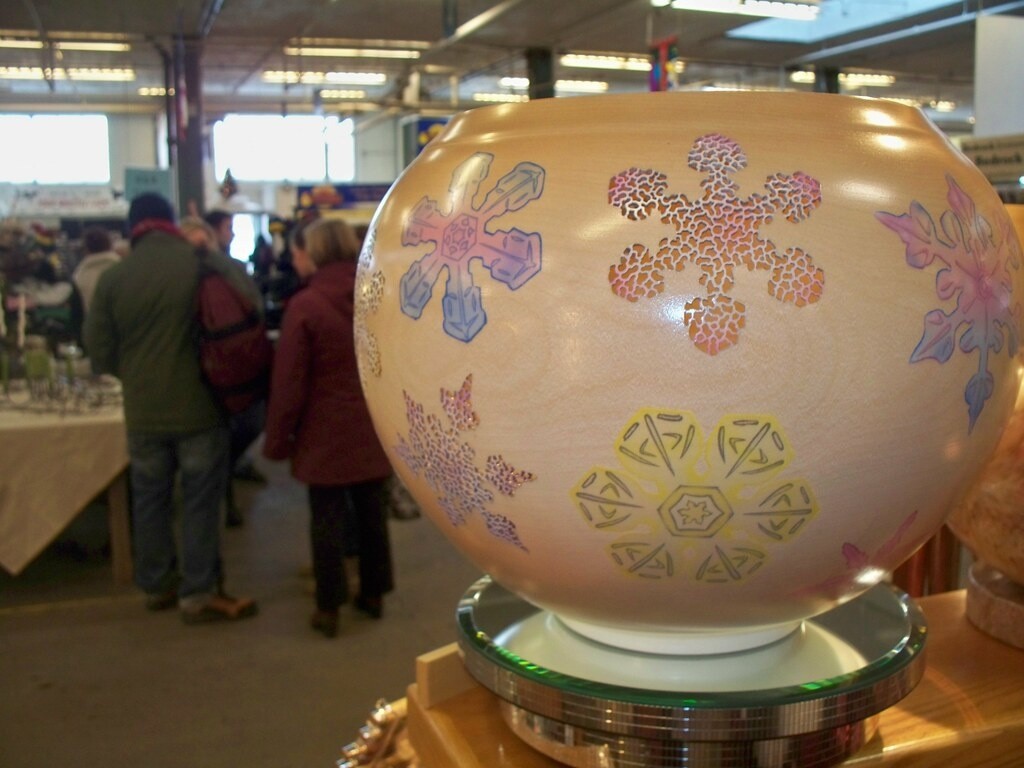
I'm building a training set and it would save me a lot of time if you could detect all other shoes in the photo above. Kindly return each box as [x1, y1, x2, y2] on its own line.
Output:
[178, 593, 256, 624]
[354, 591, 388, 619]
[311, 606, 340, 637]
[147, 592, 180, 611]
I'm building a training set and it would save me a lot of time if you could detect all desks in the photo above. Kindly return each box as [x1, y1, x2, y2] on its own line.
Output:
[0, 389, 133, 587]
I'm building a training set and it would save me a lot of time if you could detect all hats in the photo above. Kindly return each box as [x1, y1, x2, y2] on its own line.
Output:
[128, 192, 174, 227]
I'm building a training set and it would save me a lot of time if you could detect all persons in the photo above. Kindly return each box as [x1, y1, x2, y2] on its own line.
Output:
[262, 217, 422, 639]
[25, 257, 87, 360]
[248, 235, 271, 293]
[71, 225, 122, 314]
[81, 191, 258, 625]
[177, 212, 267, 528]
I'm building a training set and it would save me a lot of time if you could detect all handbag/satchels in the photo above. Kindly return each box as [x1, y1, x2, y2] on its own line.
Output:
[195, 240, 274, 413]
